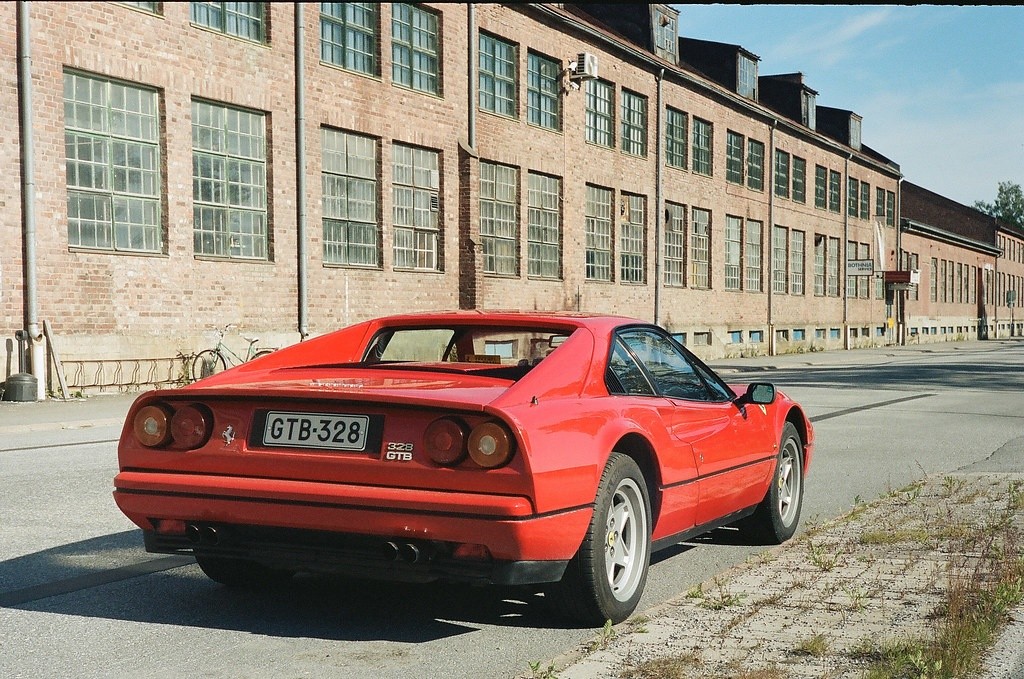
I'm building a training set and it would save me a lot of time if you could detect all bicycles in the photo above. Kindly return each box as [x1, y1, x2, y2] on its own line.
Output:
[192, 322, 280, 382]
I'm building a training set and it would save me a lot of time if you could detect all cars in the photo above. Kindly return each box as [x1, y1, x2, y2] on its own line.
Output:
[111, 309, 814, 624]
